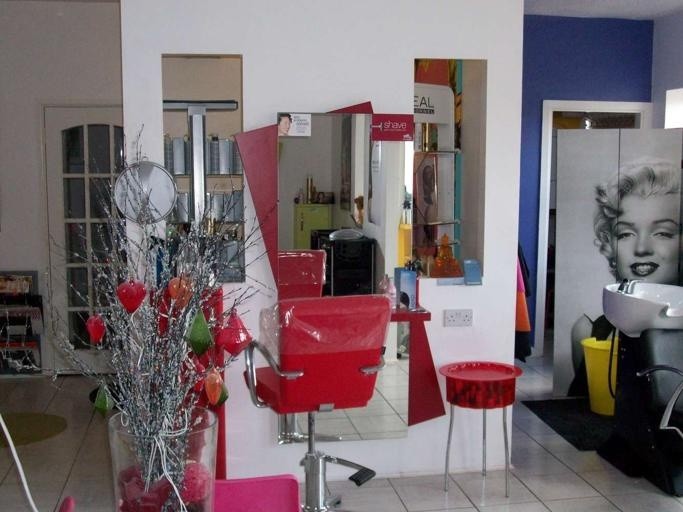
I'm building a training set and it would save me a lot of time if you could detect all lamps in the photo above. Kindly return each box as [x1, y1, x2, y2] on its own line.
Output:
[278, 249, 328, 298]
[243, 294, 395, 512]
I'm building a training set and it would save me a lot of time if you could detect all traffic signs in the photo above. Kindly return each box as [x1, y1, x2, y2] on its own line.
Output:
[445, 310, 473, 325]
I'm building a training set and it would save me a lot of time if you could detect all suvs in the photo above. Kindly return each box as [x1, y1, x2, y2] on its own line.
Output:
[580, 335, 620, 417]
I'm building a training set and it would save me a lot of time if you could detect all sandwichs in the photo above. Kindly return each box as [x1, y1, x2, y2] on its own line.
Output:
[0, 415, 75, 512]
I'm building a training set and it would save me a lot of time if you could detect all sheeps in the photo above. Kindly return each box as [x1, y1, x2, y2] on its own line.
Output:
[626, 276, 644, 293]
[349, 212, 363, 229]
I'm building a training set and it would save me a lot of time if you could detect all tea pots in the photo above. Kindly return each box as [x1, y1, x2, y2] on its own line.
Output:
[414, 58, 488, 280]
[277, 112, 415, 326]
[113, 156, 180, 223]
[161, 53, 244, 283]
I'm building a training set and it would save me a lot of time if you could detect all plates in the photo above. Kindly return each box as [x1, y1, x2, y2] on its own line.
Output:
[310, 229, 378, 297]
[165, 172, 243, 284]
[293, 203, 333, 250]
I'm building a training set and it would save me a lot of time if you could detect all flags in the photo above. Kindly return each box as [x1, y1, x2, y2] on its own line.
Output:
[107, 406, 220, 512]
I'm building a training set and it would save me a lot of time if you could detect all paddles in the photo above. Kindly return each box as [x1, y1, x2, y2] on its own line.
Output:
[1, 411, 68, 447]
[520, 396, 617, 452]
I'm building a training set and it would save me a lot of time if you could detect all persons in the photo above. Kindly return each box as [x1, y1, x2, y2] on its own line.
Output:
[566, 156, 683, 397]
[423, 164, 434, 236]
[277, 113, 292, 137]
